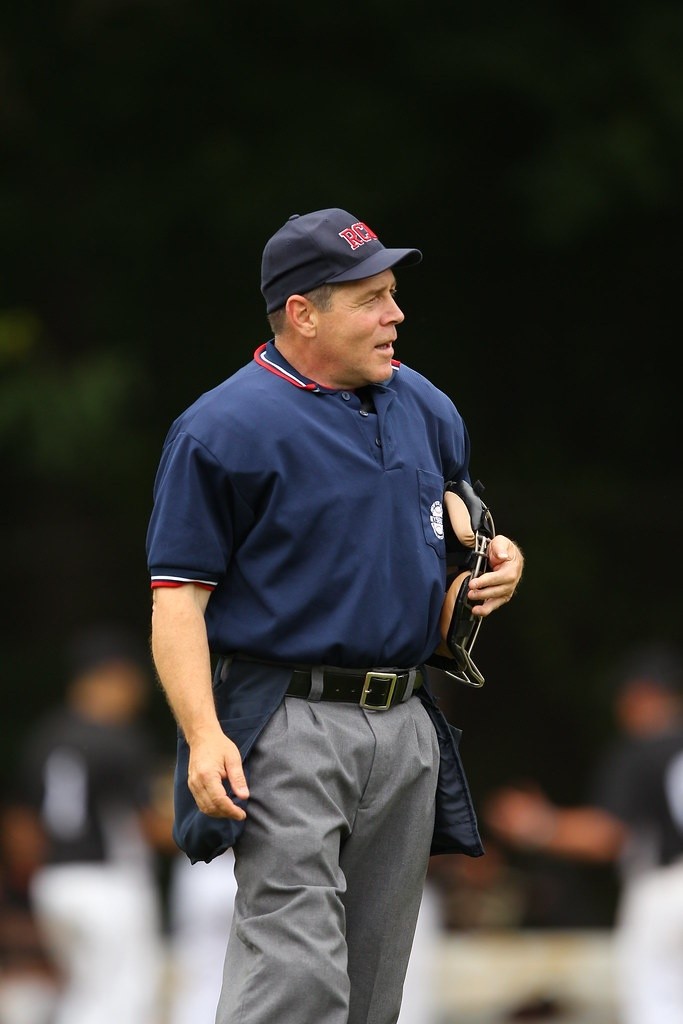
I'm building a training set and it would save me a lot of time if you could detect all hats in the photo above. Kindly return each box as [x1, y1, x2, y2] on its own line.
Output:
[260, 209, 423, 315]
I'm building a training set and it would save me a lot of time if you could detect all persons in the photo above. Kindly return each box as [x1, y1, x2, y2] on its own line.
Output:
[146, 210, 525, 1024]
[2, 624, 683, 980]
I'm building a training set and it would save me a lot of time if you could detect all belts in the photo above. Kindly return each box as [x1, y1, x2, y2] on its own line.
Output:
[284, 668, 423, 710]
